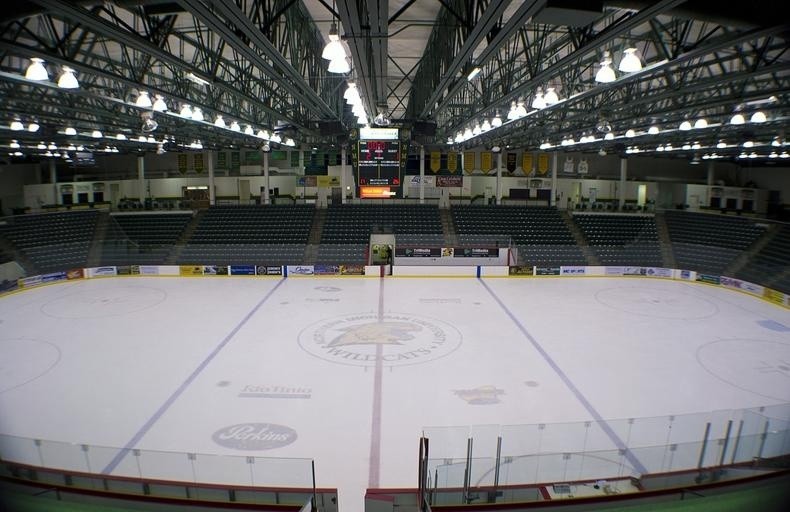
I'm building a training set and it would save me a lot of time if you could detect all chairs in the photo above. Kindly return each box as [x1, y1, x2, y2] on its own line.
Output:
[0, 203, 790, 297]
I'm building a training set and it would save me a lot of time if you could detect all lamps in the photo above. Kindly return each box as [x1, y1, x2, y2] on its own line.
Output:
[446, 84, 790, 162]
[321, 0, 347, 60]
[327, 59, 350, 74]
[343, 82, 369, 125]
[25, 57, 49, 81]
[594, 58, 616, 83]
[57, 65, 80, 89]
[618, 47, 642, 72]
[1, 92, 297, 159]
